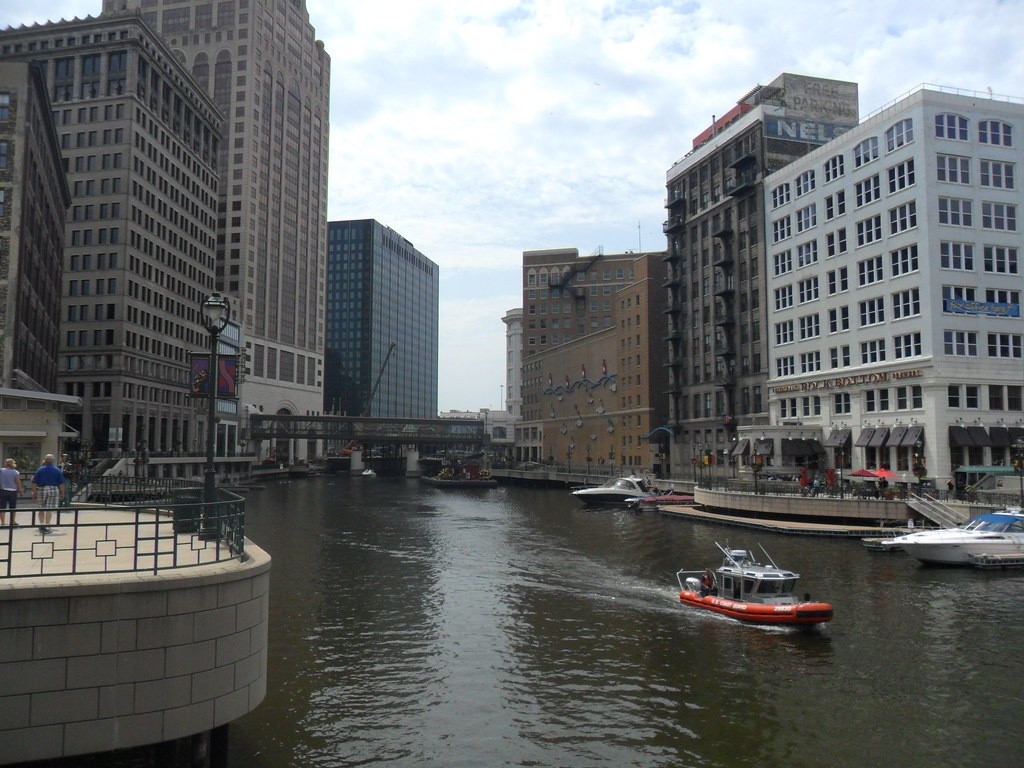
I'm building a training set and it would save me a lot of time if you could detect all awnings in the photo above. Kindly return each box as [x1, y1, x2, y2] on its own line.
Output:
[732, 438, 749, 455]
[823, 428, 851, 447]
[949, 425, 998, 447]
[782, 438, 827, 456]
[855, 426, 923, 446]
[751, 438, 774, 456]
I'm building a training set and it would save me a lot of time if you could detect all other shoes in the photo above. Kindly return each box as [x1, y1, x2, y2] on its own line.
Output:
[40, 526, 52, 534]
[12, 523, 19, 527]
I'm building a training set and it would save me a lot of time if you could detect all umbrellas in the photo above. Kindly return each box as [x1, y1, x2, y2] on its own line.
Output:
[848, 469, 879, 482]
[874, 468, 898, 478]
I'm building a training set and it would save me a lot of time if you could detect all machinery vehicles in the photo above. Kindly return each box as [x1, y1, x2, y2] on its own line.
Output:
[340, 341, 397, 457]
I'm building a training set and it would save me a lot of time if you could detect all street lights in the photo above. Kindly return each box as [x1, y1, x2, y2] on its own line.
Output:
[916, 438, 923, 497]
[500, 384, 504, 411]
[177, 427, 181, 457]
[141, 424, 145, 447]
[754, 442, 758, 494]
[586, 444, 591, 475]
[610, 444, 614, 476]
[568, 445, 571, 474]
[197, 292, 232, 541]
[840, 448, 846, 498]
[698, 443, 704, 488]
[706, 442, 712, 490]
[692, 442, 696, 482]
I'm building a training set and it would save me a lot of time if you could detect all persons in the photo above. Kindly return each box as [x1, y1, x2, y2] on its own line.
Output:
[947, 480, 955, 490]
[0, 458, 24, 527]
[812, 477, 819, 497]
[878, 476, 888, 499]
[31, 454, 65, 534]
[701, 571, 718, 598]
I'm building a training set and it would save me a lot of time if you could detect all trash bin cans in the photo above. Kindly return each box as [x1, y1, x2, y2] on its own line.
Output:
[172, 490, 201, 534]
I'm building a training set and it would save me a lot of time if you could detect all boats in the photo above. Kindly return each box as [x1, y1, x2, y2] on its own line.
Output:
[624, 498, 644, 507]
[970, 552, 1024, 569]
[570, 485, 598, 492]
[861, 537, 902, 552]
[637, 495, 695, 512]
[677, 539, 834, 624]
[571, 474, 664, 508]
[362, 469, 377, 477]
[881, 507, 1024, 565]
